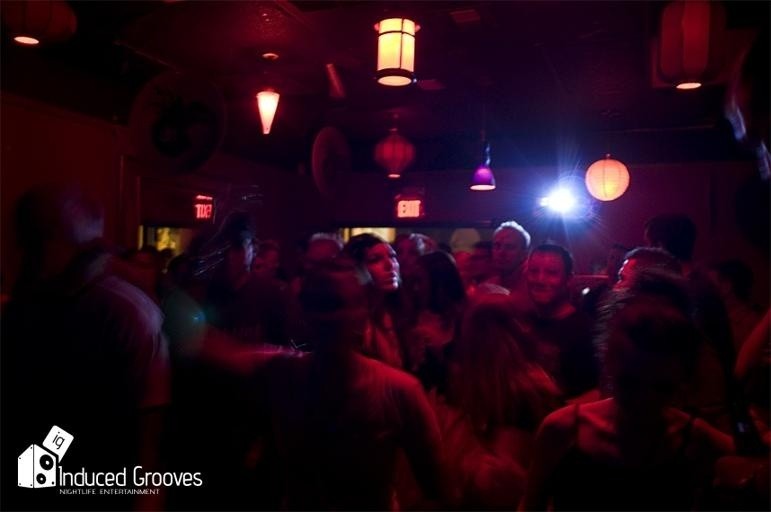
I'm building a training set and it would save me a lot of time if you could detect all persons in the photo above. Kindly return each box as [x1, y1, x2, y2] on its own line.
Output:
[1, 182, 770, 509]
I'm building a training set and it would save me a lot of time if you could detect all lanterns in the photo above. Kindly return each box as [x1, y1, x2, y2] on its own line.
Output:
[374, 128, 417, 179]
[585, 159, 630, 202]
[657, 1, 723, 91]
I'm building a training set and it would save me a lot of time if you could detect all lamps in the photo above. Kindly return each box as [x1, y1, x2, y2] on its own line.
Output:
[256, 1, 718, 204]
